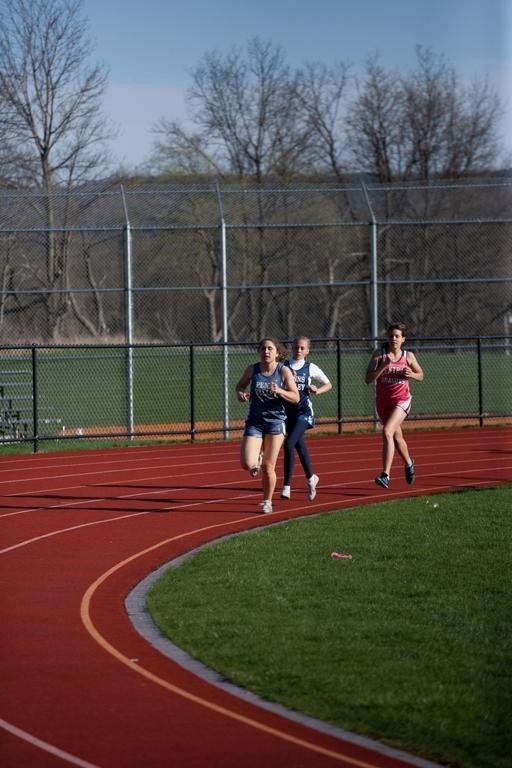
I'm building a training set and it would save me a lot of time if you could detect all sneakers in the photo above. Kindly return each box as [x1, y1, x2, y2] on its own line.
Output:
[404, 459, 415, 485]
[251, 453, 262, 477]
[374, 473, 390, 489]
[260, 499, 273, 514]
[281, 486, 290, 499]
[306, 475, 319, 502]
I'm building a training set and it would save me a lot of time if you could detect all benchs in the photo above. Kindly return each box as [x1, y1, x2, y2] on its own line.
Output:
[1, 369, 63, 440]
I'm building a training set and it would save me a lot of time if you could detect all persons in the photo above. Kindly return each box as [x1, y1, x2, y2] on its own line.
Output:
[365, 322, 425, 489]
[280, 336, 333, 501]
[235, 336, 301, 514]
